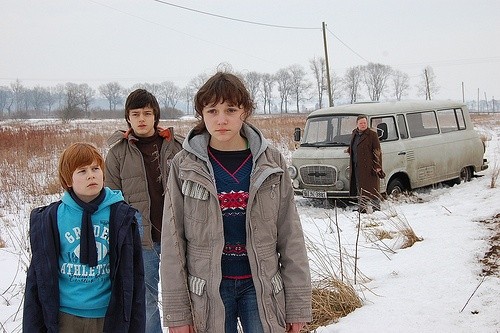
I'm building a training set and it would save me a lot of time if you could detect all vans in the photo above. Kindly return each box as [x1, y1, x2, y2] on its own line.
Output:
[286, 100, 488, 202]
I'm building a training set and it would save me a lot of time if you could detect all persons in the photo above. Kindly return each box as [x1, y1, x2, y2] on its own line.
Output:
[104, 88, 187, 333]
[22, 142, 145, 333]
[347, 115, 383, 214]
[160, 70, 313, 333]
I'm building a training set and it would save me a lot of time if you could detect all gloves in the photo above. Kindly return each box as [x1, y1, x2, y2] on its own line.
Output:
[378, 171, 386, 179]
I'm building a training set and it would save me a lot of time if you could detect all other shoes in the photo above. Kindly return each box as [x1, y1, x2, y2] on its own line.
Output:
[353, 209, 366, 213]
